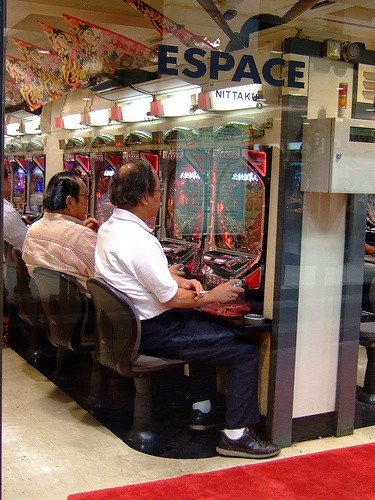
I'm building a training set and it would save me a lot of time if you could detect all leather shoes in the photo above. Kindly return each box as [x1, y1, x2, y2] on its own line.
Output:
[216, 426, 281, 459]
[189, 399, 228, 430]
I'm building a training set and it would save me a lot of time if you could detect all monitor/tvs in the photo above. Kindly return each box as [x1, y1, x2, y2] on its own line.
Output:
[12, 160, 265, 260]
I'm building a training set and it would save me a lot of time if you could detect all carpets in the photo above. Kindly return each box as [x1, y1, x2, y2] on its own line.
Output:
[68, 443, 375, 500]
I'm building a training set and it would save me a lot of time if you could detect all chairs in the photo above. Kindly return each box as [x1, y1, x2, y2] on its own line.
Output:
[2, 240, 195, 446]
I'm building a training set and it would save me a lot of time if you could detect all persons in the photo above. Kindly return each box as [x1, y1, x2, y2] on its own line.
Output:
[364, 244, 375, 255]
[21, 172, 98, 335]
[94, 159, 280, 458]
[2, 166, 29, 290]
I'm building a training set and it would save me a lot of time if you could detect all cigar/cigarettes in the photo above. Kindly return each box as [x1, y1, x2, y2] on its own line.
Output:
[193, 291, 210, 294]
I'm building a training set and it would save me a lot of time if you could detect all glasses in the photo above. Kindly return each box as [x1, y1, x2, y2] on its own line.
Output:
[77, 193, 92, 199]
[152, 187, 164, 194]
